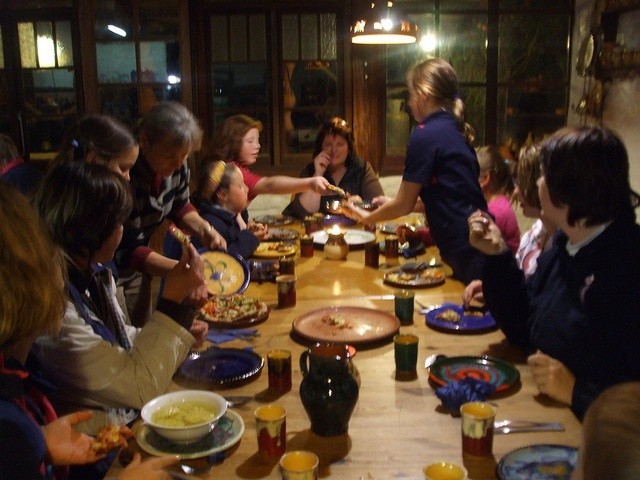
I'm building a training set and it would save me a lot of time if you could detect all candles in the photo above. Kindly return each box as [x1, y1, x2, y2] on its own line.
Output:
[323, 229, 349, 260]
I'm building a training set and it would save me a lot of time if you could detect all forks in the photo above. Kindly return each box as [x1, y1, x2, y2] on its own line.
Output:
[226, 401, 247, 410]
[152, 461, 214, 477]
[493, 416, 547, 428]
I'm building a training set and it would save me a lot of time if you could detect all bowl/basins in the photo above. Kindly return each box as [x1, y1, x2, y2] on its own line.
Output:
[141, 389, 228, 445]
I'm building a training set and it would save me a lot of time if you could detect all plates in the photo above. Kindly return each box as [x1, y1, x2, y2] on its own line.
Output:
[426, 304, 497, 332]
[198, 302, 268, 329]
[255, 239, 298, 259]
[496, 443, 582, 479]
[429, 354, 521, 394]
[135, 410, 243, 459]
[382, 238, 425, 257]
[197, 246, 249, 295]
[325, 200, 377, 214]
[261, 227, 300, 239]
[178, 346, 264, 385]
[310, 227, 375, 249]
[292, 305, 400, 346]
[383, 270, 447, 289]
[252, 214, 293, 227]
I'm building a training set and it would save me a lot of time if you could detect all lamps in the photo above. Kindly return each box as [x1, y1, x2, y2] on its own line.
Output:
[347, 0, 421, 46]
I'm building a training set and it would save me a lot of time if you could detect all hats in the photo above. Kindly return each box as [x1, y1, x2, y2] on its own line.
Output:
[200, 160, 226, 199]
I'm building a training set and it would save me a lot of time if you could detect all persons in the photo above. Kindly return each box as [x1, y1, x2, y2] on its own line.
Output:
[462, 132, 558, 310]
[0, 133, 39, 196]
[467, 124, 640, 419]
[110, 100, 227, 328]
[395, 145, 521, 257]
[567, 382, 640, 480]
[278, 117, 384, 221]
[0, 185, 137, 480]
[346, 56, 499, 283]
[155, 160, 268, 323]
[197, 114, 339, 203]
[30, 158, 209, 411]
[47, 114, 141, 182]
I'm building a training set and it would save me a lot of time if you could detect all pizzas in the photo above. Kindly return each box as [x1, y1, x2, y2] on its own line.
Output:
[387, 265, 446, 283]
[435, 306, 484, 324]
[199, 293, 263, 324]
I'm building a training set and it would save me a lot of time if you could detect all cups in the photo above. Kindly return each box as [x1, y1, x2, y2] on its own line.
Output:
[280, 450, 318, 479]
[280, 255, 295, 273]
[394, 333, 420, 381]
[300, 233, 315, 258]
[268, 350, 291, 394]
[460, 401, 497, 455]
[425, 462, 466, 479]
[276, 274, 297, 308]
[385, 234, 399, 267]
[304, 215, 316, 233]
[394, 289, 416, 325]
[255, 405, 287, 459]
[317, 213, 324, 226]
[364, 243, 380, 268]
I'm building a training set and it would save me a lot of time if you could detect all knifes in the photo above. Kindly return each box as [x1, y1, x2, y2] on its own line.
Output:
[498, 428, 567, 434]
[223, 393, 253, 403]
